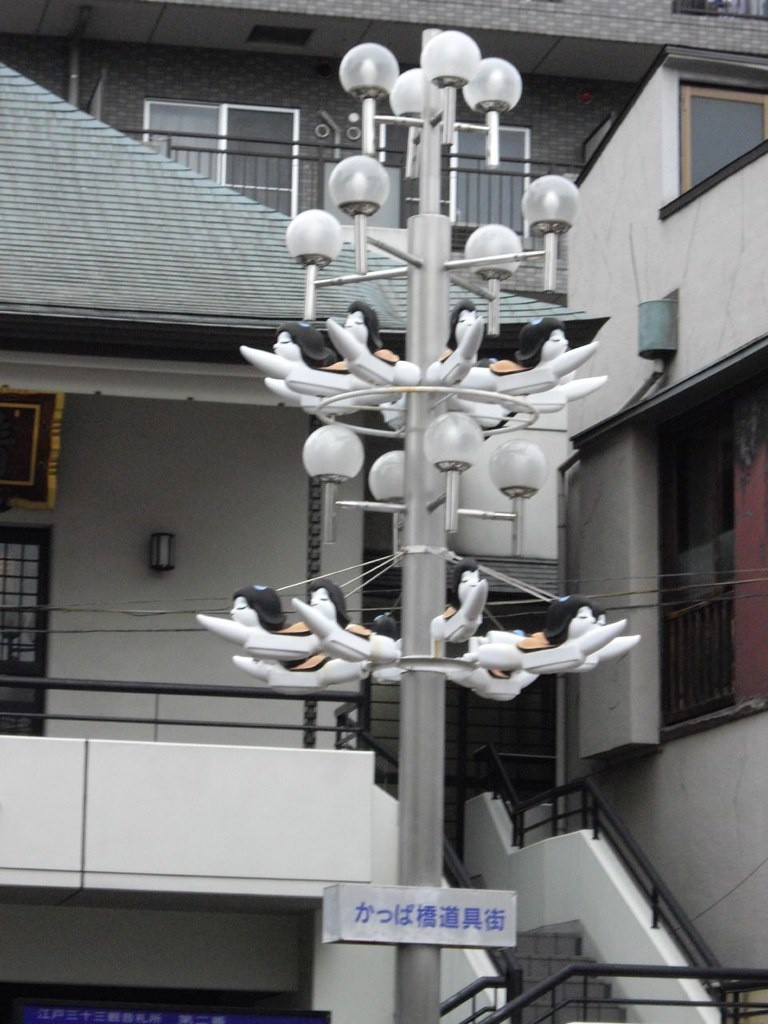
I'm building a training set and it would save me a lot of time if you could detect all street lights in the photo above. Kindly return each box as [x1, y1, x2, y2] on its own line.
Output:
[198, 28, 641, 1023]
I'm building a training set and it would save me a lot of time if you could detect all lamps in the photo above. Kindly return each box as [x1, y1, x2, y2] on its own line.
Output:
[149, 533, 176, 571]
